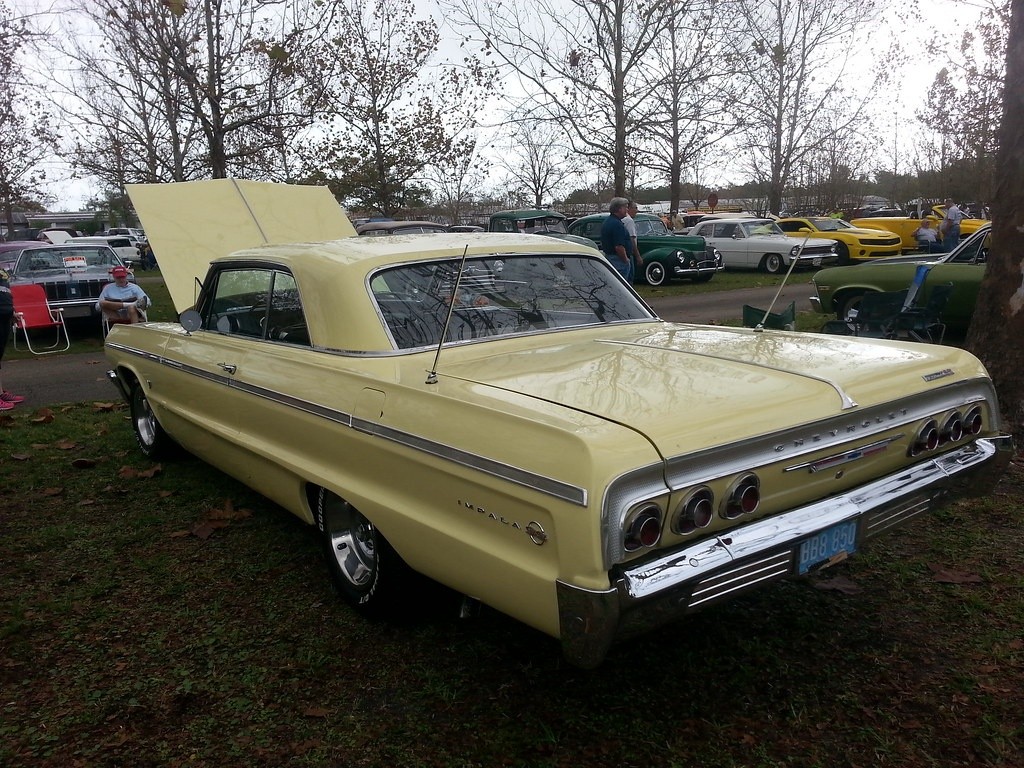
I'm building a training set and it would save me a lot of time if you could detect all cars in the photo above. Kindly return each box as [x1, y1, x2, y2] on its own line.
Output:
[0, 226, 149, 323]
[6, 242, 137, 342]
[103, 177, 1017, 669]
[809, 220, 994, 340]
[344, 193, 993, 287]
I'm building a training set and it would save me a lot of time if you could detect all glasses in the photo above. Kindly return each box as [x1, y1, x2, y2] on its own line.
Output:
[630, 206, 638, 210]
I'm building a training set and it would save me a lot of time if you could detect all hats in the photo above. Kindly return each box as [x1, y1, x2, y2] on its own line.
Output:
[671, 210, 677, 214]
[112, 266, 127, 277]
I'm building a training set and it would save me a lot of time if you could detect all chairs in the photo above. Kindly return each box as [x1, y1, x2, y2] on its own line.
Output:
[276, 324, 311, 346]
[10, 284, 70, 356]
[228, 312, 264, 337]
[101, 306, 148, 341]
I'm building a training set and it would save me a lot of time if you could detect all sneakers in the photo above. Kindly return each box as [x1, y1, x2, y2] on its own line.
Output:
[136, 296, 147, 311]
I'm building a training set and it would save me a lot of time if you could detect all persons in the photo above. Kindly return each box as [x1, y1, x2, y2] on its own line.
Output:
[95, 266, 152, 325]
[671, 210, 685, 232]
[830, 208, 843, 220]
[0, 267, 26, 410]
[600, 197, 631, 291]
[657, 213, 671, 231]
[940, 197, 962, 254]
[619, 199, 643, 290]
[910, 219, 946, 256]
